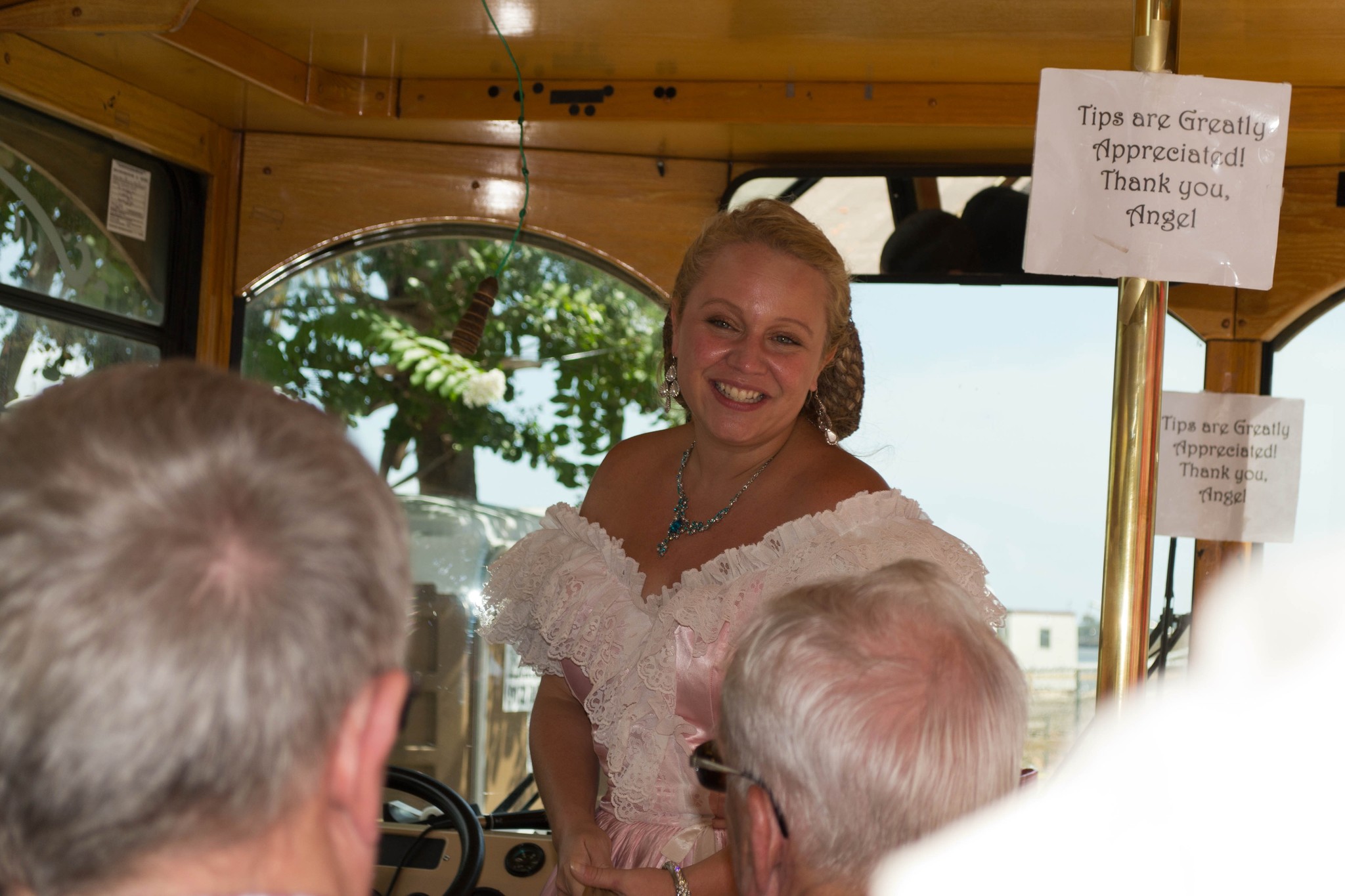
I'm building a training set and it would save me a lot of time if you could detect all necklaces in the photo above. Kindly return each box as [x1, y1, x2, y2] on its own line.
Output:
[656, 439, 777, 558]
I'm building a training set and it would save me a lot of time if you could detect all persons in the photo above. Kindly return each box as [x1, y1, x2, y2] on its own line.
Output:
[0, 364, 417, 896]
[880, 210, 982, 276]
[688, 558, 1026, 896]
[962, 188, 1028, 271]
[480, 196, 1008, 896]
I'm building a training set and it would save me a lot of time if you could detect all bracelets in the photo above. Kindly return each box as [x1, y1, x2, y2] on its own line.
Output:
[662, 861, 691, 896]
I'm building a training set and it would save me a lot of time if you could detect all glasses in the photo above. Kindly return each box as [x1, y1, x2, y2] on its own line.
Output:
[690, 740, 789, 838]
